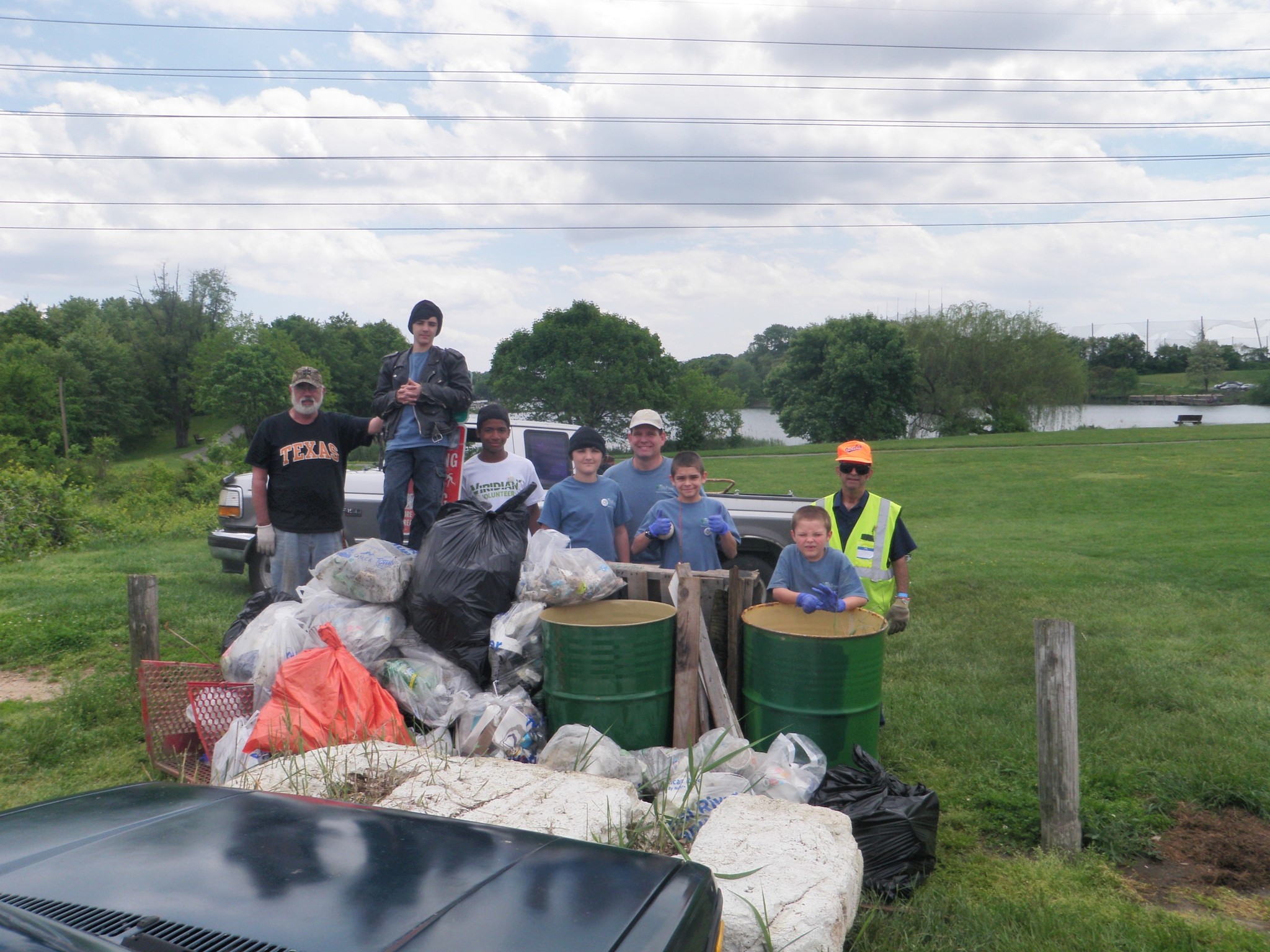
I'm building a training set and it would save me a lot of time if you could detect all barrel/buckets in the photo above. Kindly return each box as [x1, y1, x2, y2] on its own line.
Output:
[542, 591, 681, 758]
[738, 593, 887, 785]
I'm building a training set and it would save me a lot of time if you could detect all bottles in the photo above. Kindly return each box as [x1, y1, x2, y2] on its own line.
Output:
[529, 635, 542, 660]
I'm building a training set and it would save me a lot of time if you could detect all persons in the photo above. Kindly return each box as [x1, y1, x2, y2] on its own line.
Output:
[461, 404, 546, 539]
[630, 451, 741, 571]
[766, 505, 869, 613]
[810, 440, 917, 636]
[602, 409, 706, 565]
[536, 426, 633, 563]
[243, 365, 385, 597]
[368, 299, 473, 550]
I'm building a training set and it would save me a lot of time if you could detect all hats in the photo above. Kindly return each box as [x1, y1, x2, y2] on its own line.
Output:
[291, 366, 322, 388]
[477, 404, 511, 429]
[835, 440, 872, 466]
[629, 409, 664, 432]
[408, 299, 443, 336]
[569, 426, 607, 458]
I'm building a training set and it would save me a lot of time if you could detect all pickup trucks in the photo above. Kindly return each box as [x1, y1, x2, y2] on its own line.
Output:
[206, 408, 828, 609]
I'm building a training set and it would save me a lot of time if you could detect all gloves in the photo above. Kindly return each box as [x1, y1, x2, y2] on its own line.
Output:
[883, 598, 909, 636]
[255, 522, 276, 557]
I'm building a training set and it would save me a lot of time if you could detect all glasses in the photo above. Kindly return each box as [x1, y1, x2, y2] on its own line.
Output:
[838, 463, 869, 475]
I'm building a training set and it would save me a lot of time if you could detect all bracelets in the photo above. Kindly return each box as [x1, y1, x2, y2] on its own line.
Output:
[897, 593, 909, 598]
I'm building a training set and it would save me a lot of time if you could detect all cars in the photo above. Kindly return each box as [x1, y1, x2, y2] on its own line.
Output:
[1214, 380, 1256, 391]
[0, 779, 727, 951]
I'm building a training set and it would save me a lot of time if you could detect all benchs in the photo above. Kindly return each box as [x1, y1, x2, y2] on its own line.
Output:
[1173, 414, 1203, 426]
[194, 433, 205, 444]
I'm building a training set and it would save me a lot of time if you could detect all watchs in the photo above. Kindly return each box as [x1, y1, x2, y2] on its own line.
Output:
[645, 529, 655, 540]
